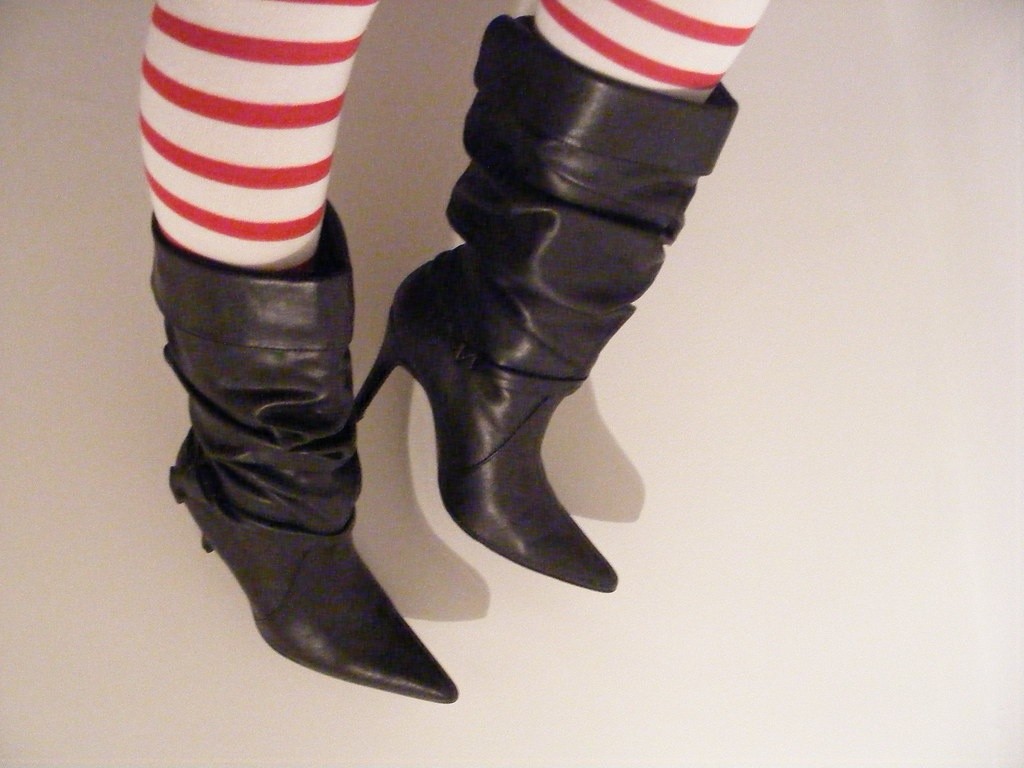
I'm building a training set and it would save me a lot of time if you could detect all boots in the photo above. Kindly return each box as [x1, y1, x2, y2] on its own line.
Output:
[149, 201, 461, 704]
[350, 14, 740, 594]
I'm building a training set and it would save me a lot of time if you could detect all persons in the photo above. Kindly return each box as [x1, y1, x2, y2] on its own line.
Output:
[137, 0, 773, 706]
